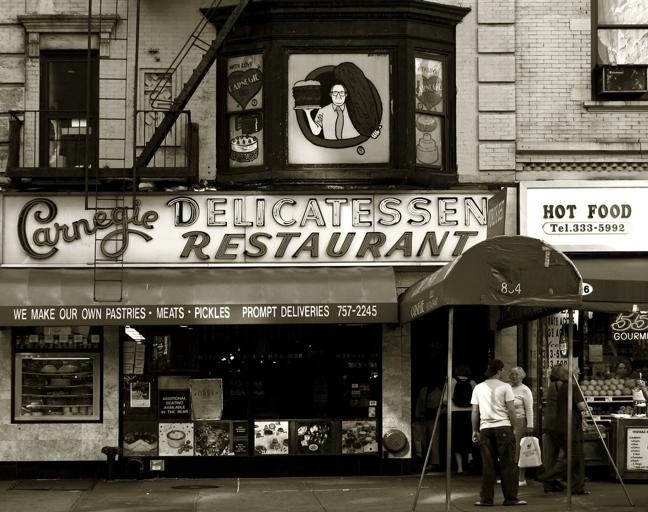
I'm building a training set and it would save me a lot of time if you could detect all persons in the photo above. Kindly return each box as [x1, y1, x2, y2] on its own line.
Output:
[412, 358, 591, 507]
[613, 359, 633, 377]
[303, 84, 383, 139]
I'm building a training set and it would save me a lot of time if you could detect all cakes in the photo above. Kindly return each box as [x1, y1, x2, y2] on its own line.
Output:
[230, 135, 259, 162]
[293, 79, 322, 108]
[416, 114, 437, 132]
[235, 112, 262, 135]
[416, 133, 439, 164]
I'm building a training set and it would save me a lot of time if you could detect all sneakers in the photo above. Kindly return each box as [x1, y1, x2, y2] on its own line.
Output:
[503, 499, 527, 505]
[426, 464, 439, 470]
[572, 489, 590, 496]
[519, 479, 527, 487]
[544, 481, 566, 493]
[469, 459, 474, 465]
[475, 501, 494, 506]
[455, 472, 463, 475]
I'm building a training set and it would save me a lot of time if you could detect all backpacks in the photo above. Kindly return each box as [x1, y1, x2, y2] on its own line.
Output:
[453, 376, 473, 407]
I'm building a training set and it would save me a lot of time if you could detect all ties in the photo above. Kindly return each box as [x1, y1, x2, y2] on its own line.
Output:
[335, 107, 344, 140]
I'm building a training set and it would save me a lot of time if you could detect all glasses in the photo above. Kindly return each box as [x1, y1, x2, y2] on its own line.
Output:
[331, 91, 346, 96]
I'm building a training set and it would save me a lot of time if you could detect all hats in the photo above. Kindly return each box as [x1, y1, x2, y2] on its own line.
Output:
[484, 359, 504, 377]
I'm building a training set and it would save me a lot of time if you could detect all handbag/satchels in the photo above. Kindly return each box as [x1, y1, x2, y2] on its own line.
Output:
[424, 408, 438, 419]
[517, 437, 542, 467]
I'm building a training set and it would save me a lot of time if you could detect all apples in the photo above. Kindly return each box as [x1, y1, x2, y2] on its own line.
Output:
[580, 379, 625, 396]
